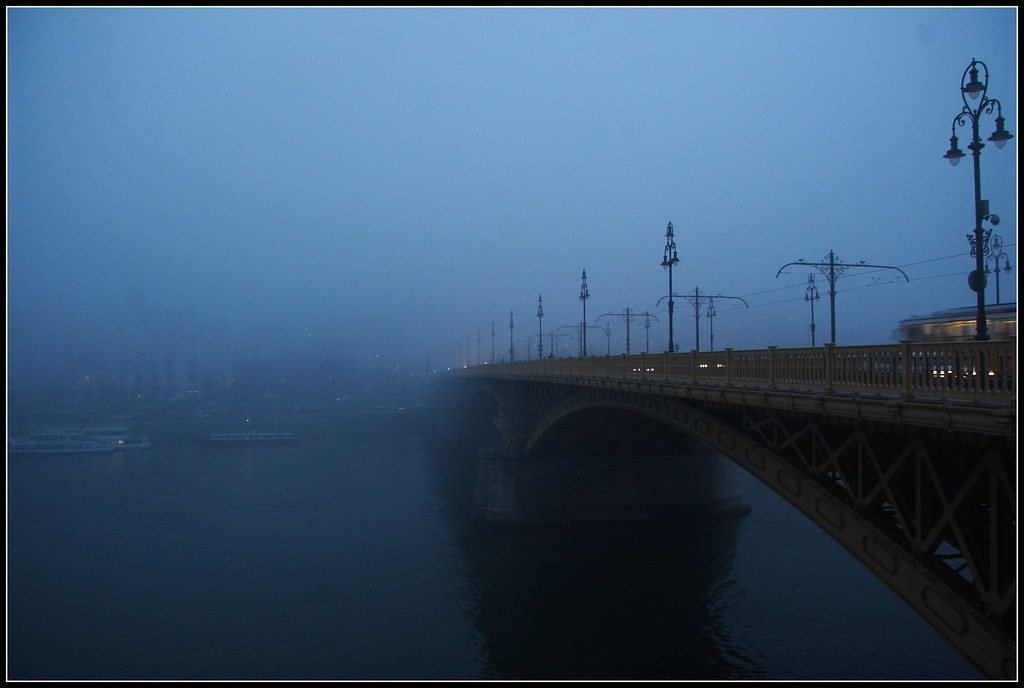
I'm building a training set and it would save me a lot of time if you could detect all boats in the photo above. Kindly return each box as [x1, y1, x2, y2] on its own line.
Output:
[8, 432, 153, 454]
[192, 431, 297, 448]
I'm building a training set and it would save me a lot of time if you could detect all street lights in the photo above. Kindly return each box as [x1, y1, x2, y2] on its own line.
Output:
[579, 270, 589, 357]
[491, 322, 495, 364]
[509, 310, 514, 361]
[467, 328, 480, 367]
[660, 220, 680, 351]
[944, 56, 1014, 395]
[536, 294, 544, 359]
